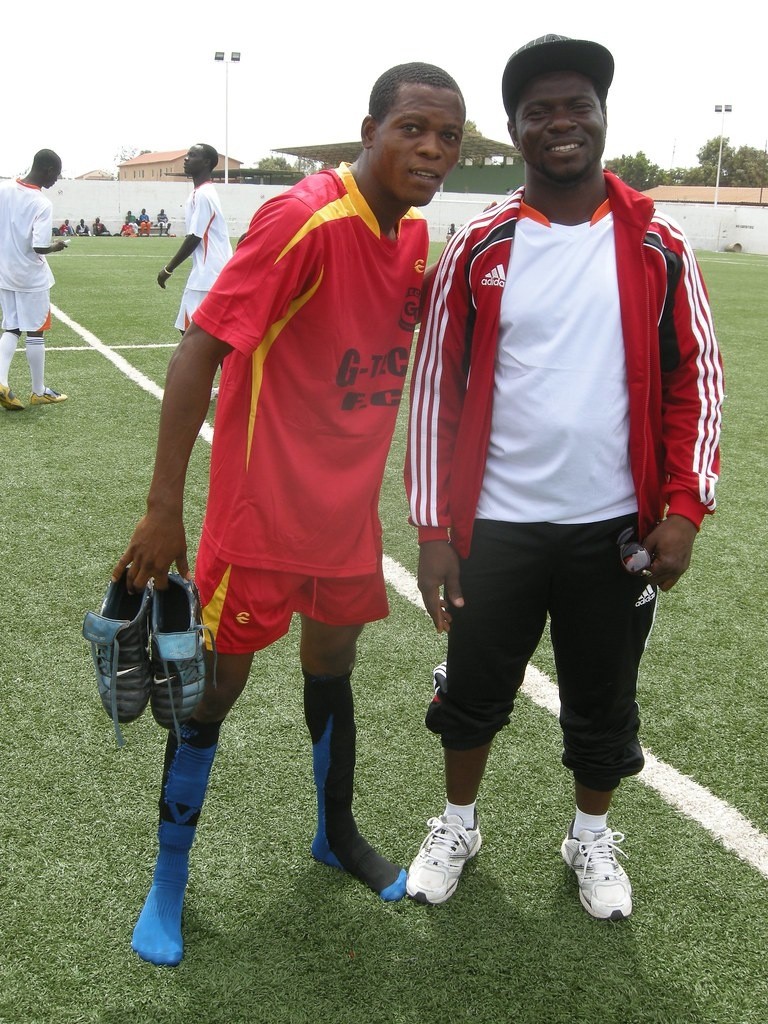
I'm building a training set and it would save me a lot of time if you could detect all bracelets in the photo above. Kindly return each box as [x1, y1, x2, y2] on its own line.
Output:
[163, 266, 174, 274]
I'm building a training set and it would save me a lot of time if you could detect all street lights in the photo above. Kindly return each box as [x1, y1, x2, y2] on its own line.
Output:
[713, 104, 732, 205]
[213, 50, 241, 182]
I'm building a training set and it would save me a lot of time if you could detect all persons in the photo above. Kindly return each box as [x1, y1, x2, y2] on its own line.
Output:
[120, 208, 172, 237]
[0, 148, 70, 411]
[59, 219, 76, 236]
[110, 59, 467, 968]
[447, 224, 456, 235]
[405, 31, 726, 927]
[93, 217, 112, 236]
[76, 219, 92, 236]
[155, 144, 235, 394]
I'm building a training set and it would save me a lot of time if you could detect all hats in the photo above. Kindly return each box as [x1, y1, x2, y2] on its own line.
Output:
[502, 34, 614, 121]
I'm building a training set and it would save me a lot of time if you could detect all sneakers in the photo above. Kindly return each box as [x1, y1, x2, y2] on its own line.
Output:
[211, 387, 219, 400]
[31, 388, 68, 403]
[0, 384, 24, 410]
[83, 568, 217, 747]
[406, 807, 482, 903]
[561, 818, 633, 919]
